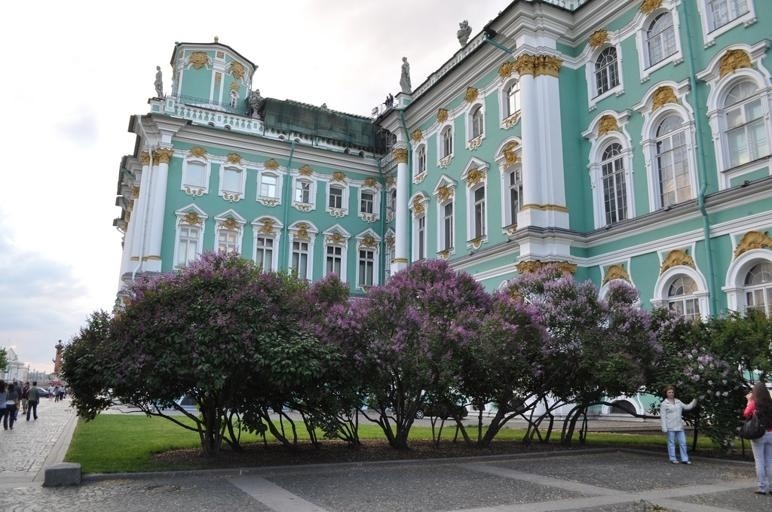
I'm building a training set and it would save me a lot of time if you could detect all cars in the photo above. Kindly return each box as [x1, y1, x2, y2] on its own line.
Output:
[48, 387, 57, 397]
[36, 386, 51, 398]
[415, 394, 470, 421]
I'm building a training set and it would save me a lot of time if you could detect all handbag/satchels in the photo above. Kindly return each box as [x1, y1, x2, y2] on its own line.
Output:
[739, 414, 766, 440]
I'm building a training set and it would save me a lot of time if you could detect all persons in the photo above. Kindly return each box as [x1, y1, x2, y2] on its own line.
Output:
[741, 380, 772, 496]
[658, 384, 699, 465]
[1, 377, 72, 428]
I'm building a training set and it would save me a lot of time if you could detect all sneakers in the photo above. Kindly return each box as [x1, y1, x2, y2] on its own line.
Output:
[671, 460, 680, 464]
[755, 489, 766, 495]
[682, 461, 692, 465]
